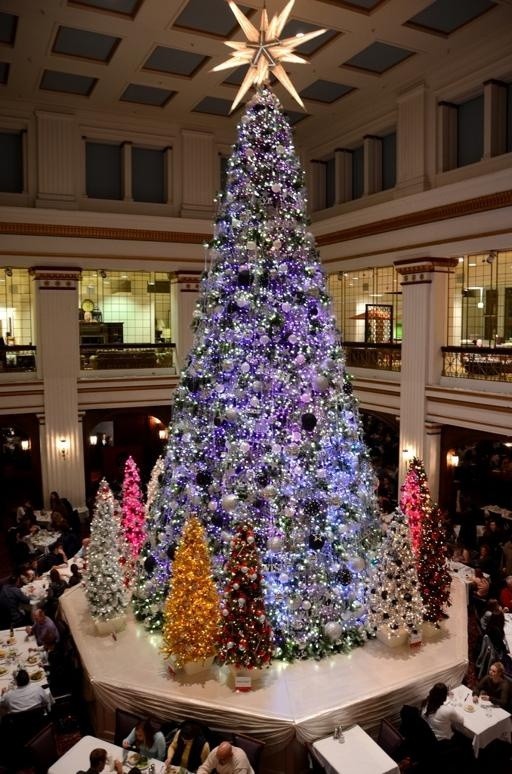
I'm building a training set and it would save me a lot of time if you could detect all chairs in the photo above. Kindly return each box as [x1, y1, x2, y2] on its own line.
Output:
[113, 707, 144, 747]
[378, 717, 405, 760]
[233, 732, 265, 773]
[303, 741, 324, 773]
[24, 722, 58, 774]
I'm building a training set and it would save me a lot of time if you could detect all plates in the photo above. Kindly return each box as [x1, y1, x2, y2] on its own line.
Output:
[444, 690, 496, 720]
[104, 754, 188, 774]
[0, 629, 44, 695]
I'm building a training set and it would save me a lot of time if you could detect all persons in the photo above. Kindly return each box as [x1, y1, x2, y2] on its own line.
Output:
[95, 436, 112, 448]
[76, 714, 255, 774]
[2, 423, 28, 463]
[1, 492, 82, 659]
[359, 415, 510, 772]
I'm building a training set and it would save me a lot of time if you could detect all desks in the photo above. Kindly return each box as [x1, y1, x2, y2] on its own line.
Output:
[6, 355, 36, 369]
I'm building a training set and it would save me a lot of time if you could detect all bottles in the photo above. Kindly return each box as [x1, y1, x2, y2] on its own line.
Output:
[8, 626, 14, 636]
[465, 574, 469, 580]
[334, 723, 343, 740]
[42, 583, 45, 590]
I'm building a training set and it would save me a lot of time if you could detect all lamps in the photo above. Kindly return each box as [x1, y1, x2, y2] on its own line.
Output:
[210, 0, 331, 115]
[21, 437, 32, 452]
[451, 454, 459, 468]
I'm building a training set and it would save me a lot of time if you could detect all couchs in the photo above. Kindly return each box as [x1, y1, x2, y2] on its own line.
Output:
[89, 350, 158, 369]
